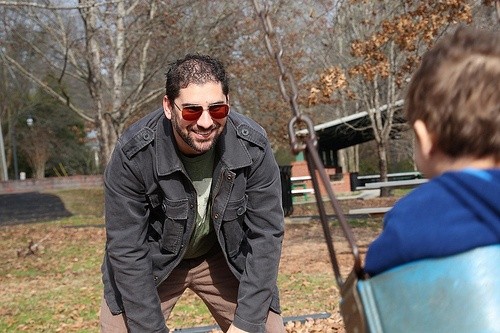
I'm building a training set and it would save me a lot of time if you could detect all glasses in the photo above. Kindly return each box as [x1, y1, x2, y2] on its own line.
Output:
[172, 95, 230, 122]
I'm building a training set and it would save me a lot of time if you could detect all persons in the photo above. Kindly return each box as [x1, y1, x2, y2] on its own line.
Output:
[100, 52, 283, 333]
[364, 22, 500, 279]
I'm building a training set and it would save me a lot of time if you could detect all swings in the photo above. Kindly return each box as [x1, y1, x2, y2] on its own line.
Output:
[247, 0, 500, 333]
